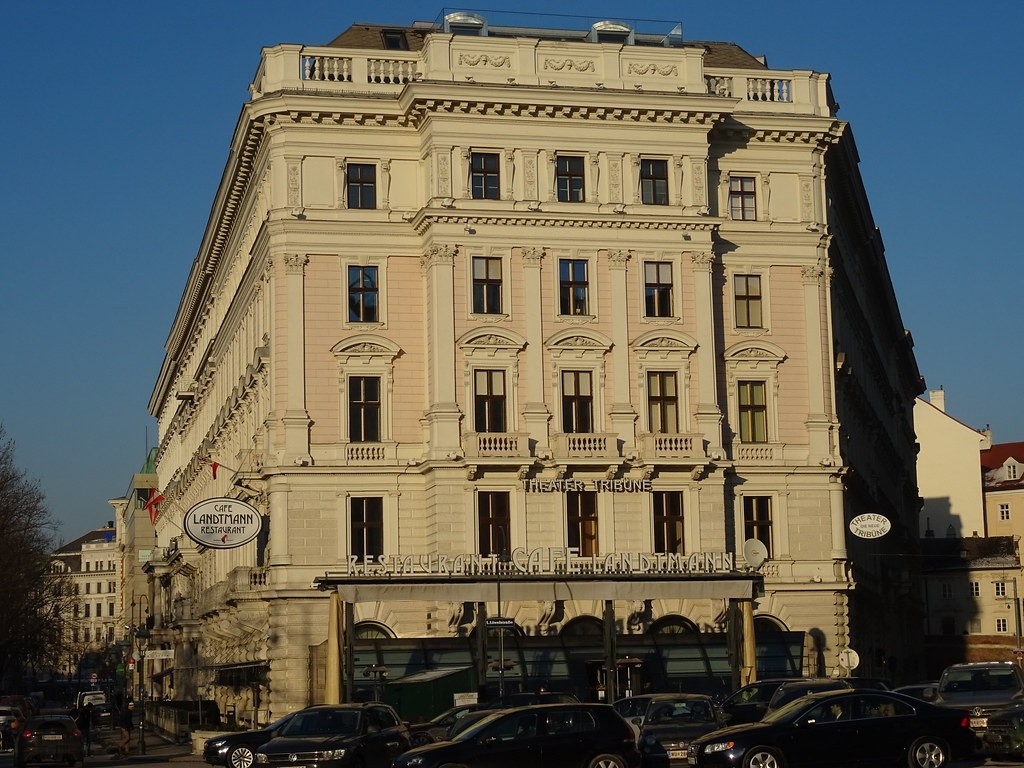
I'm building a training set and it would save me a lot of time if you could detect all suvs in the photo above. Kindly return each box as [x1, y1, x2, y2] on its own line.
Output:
[934, 659, 1024, 760]
[763, 678, 853, 730]
[249, 699, 411, 768]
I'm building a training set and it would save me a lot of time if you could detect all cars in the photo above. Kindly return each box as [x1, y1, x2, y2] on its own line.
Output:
[392, 703, 642, 767]
[886, 682, 941, 705]
[202, 709, 303, 768]
[501, 691, 582, 709]
[91, 703, 123, 729]
[608, 692, 659, 748]
[12, 712, 87, 768]
[0, 692, 41, 753]
[631, 694, 722, 768]
[686, 688, 974, 768]
[399, 703, 504, 746]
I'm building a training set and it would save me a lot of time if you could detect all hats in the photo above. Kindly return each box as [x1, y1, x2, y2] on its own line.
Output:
[129, 702, 135, 707]
[87, 702, 93, 708]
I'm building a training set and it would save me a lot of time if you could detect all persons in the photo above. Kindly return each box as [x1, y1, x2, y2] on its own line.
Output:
[880, 705, 895, 717]
[78, 702, 95, 758]
[830, 702, 848, 720]
[117, 727, 130, 760]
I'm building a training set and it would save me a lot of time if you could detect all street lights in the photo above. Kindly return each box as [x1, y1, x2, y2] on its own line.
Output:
[133, 621, 154, 757]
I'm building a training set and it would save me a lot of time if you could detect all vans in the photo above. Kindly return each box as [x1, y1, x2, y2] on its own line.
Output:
[717, 679, 893, 731]
[77, 689, 108, 711]
